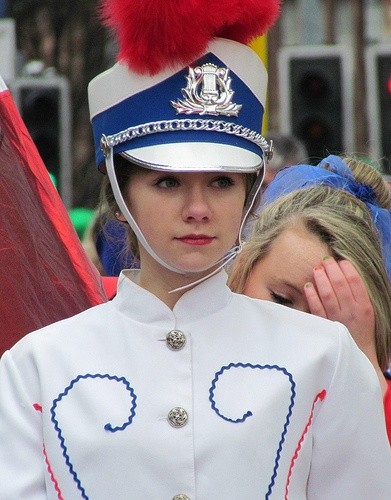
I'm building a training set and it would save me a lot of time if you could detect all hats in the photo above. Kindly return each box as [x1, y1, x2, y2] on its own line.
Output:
[87, 1, 274, 174]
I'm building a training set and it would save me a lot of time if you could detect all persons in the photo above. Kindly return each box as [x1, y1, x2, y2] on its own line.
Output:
[227, 155, 391, 400]
[0, 36, 391, 500]
